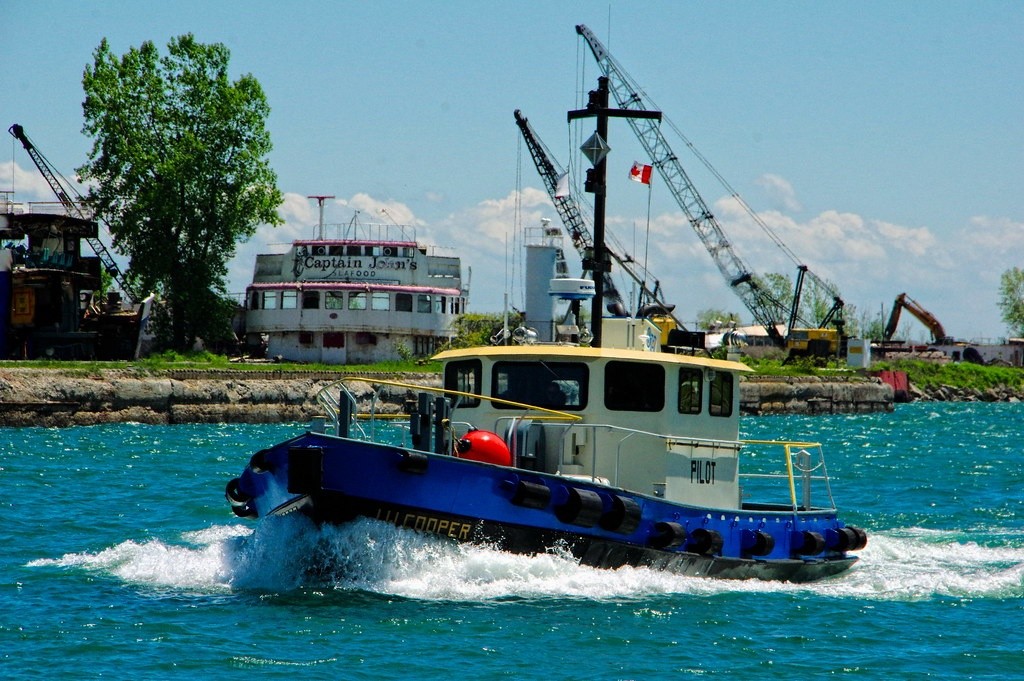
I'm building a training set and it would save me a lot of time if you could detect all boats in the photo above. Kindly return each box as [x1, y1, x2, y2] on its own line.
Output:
[244, 196, 468, 369]
[222, 72, 872, 595]
[0, 213, 142, 363]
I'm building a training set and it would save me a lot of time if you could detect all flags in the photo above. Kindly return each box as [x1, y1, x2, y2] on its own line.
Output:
[627, 160, 653, 185]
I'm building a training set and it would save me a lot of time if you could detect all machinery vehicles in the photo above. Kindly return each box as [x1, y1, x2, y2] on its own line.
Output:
[511, 104, 678, 349]
[575, 19, 853, 354]
[879, 291, 967, 348]
